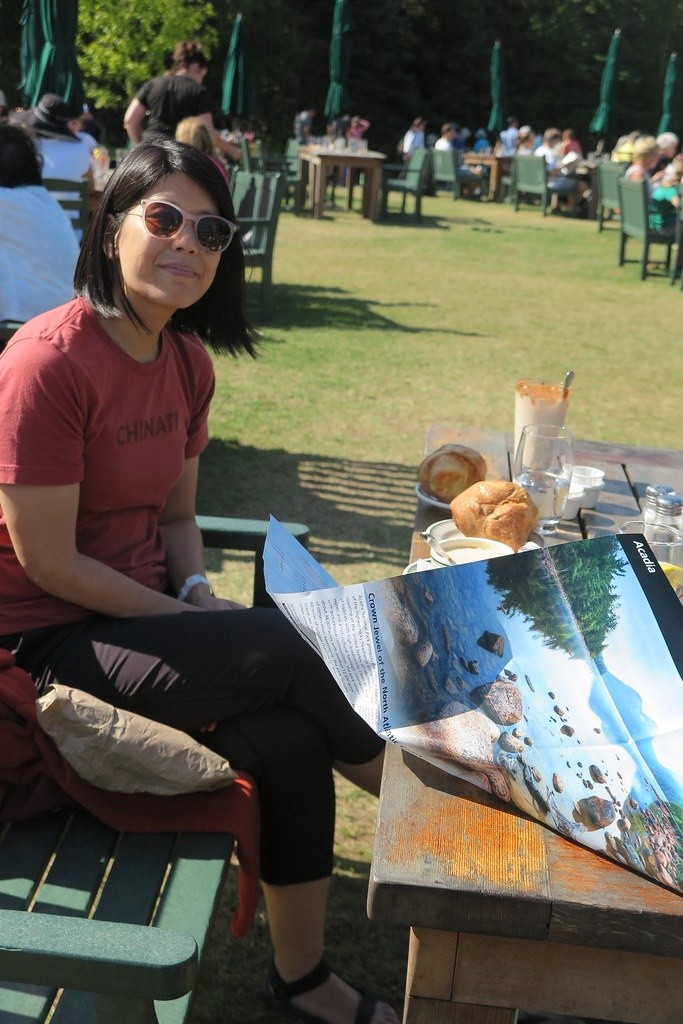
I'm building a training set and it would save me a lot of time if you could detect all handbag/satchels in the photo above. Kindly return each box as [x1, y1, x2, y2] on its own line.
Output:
[34, 682, 241, 795]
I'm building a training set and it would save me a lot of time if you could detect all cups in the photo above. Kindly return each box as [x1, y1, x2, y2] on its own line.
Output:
[619, 521, 683, 599]
[310, 136, 368, 154]
[553, 464, 606, 521]
[514, 378, 571, 478]
[514, 425, 575, 535]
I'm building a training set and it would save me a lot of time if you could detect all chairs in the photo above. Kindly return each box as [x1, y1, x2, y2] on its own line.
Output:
[41, 178, 89, 232]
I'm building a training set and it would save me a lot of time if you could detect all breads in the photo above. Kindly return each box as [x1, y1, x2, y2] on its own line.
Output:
[418, 443, 486, 504]
[449, 480, 540, 555]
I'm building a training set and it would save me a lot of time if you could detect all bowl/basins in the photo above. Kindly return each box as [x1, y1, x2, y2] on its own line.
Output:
[431, 537, 515, 568]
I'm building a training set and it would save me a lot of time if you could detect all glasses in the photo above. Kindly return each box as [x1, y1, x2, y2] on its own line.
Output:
[117, 198, 240, 255]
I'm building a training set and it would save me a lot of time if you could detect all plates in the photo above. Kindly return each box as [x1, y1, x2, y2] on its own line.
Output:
[422, 516, 546, 560]
[402, 557, 431, 578]
[414, 482, 455, 515]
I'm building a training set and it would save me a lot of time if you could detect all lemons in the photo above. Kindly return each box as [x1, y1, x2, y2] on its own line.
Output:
[661, 565, 683, 591]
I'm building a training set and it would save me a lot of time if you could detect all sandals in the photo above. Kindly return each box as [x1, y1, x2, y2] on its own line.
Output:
[265, 960, 402, 1024]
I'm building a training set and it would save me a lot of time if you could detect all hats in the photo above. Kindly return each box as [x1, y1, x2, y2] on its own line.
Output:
[9, 93, 82, 142]
[633, 135, 658, 155]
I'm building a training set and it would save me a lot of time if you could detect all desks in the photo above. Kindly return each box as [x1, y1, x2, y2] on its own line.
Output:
[368, 425, 683, 1024]
[296, 145, 386, 222]
[462, 153, 513, 203]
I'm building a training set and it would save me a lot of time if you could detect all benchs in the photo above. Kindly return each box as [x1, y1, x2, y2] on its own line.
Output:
[0, 516, 312, 1023]
[227, 139, 306, 323]
[381, 148, 683, 290]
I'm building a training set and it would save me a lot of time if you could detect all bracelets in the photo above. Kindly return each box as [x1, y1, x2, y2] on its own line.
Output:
[176, 573, 216, 601]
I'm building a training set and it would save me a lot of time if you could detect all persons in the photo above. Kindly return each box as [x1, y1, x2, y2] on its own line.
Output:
[123, 42, 243, 162]
[1, 139, 403, 1024]
[176, 116, 228, 186]
[493, 117, 519, 175]
[434, 123, 480, 202]
[0, 94, 110, 354]
[516, 125, 683, 239]
[293, 108, 370, 187]
[403, 117, 425, 165]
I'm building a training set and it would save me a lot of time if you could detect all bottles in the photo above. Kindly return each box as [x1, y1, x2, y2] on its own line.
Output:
[642, 485, 676, 545]
[654, 495, 683, 537]
